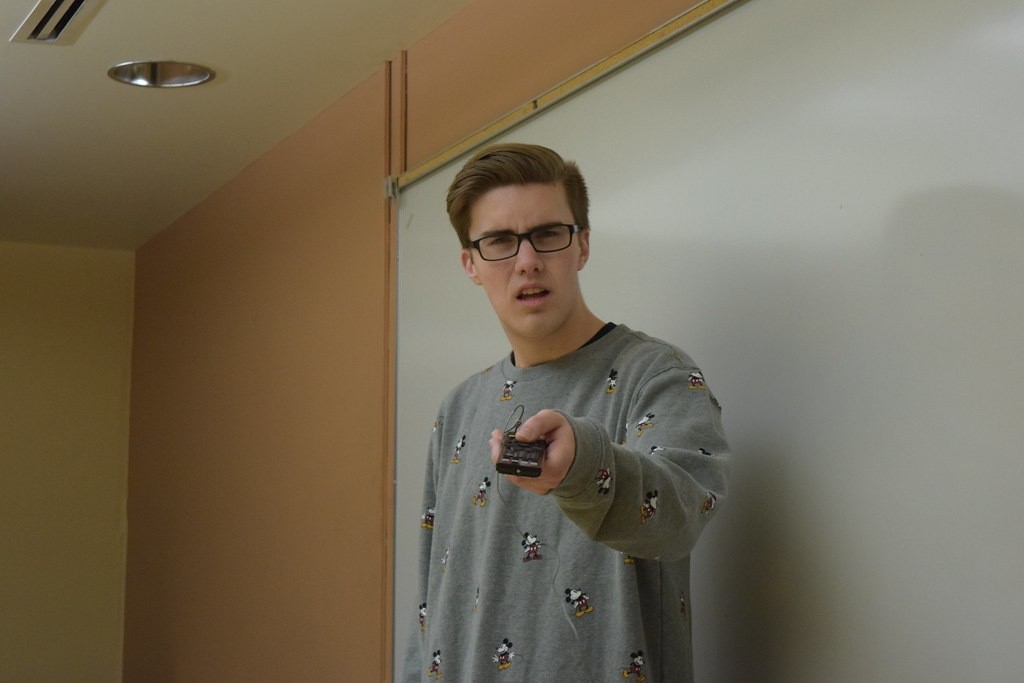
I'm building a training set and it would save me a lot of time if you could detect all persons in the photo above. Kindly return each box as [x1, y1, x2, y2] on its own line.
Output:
[402, 144, 734, 683]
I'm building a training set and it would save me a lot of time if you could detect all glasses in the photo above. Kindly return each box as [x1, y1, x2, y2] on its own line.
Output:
[467, 224, 582, 261]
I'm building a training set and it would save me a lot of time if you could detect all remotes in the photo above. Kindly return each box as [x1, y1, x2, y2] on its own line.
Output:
[495, 431, 547, 477]
